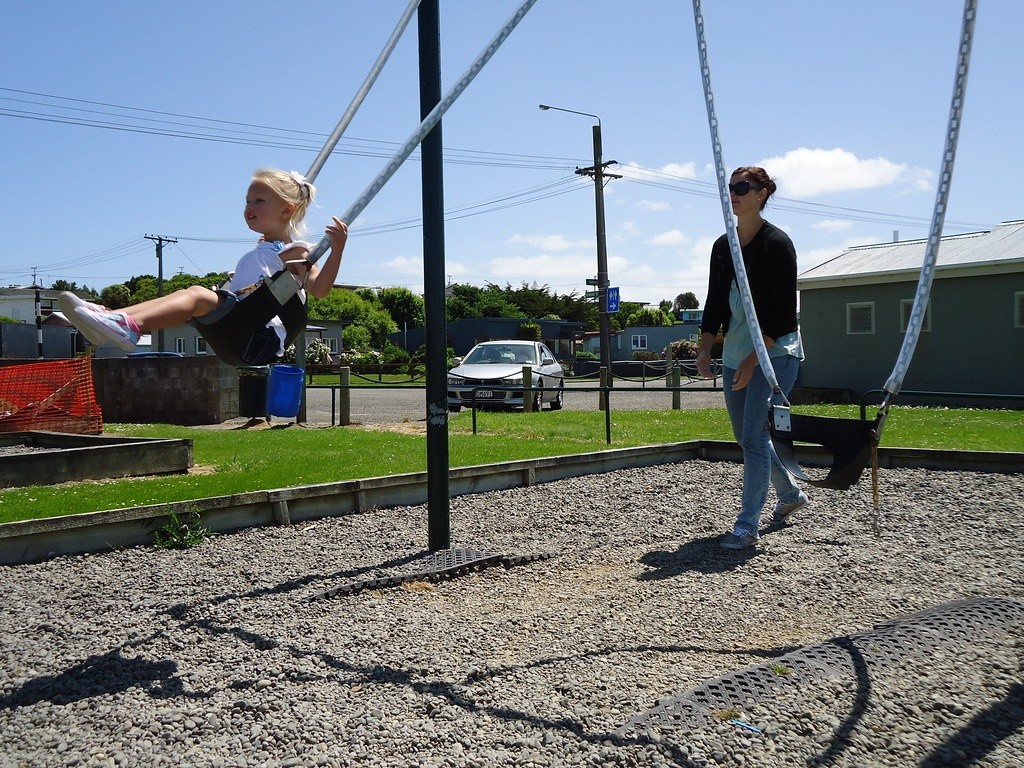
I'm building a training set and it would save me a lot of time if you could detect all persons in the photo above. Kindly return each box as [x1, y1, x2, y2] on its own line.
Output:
[58, 170, 348, 351]
[694, 166, 808, 548]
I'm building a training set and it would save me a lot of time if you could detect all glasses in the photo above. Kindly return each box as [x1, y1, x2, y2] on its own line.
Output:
[729, 181, 763, 195]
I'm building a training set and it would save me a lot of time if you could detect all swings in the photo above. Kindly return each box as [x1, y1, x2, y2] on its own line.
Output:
[691, 0, 980, 494]
[184, 1, 540, 368]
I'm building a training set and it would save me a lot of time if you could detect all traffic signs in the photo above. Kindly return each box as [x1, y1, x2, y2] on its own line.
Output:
[607, 287, 620, 313]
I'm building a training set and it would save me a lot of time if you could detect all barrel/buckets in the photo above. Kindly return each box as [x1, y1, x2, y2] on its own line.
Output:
[266, 365, 305, 417]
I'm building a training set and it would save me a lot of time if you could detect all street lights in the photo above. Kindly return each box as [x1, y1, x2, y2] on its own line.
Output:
[538, 104, 614, 389]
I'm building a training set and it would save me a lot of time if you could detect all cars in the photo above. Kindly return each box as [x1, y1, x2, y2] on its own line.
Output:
[447, 340, 564, 412]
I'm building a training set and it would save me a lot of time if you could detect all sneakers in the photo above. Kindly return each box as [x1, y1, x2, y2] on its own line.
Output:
[58, 291, 107, 346]
[74, 307, 143, 352]
[721, 533, 760, 549]
[761, 490, 808, 524]
